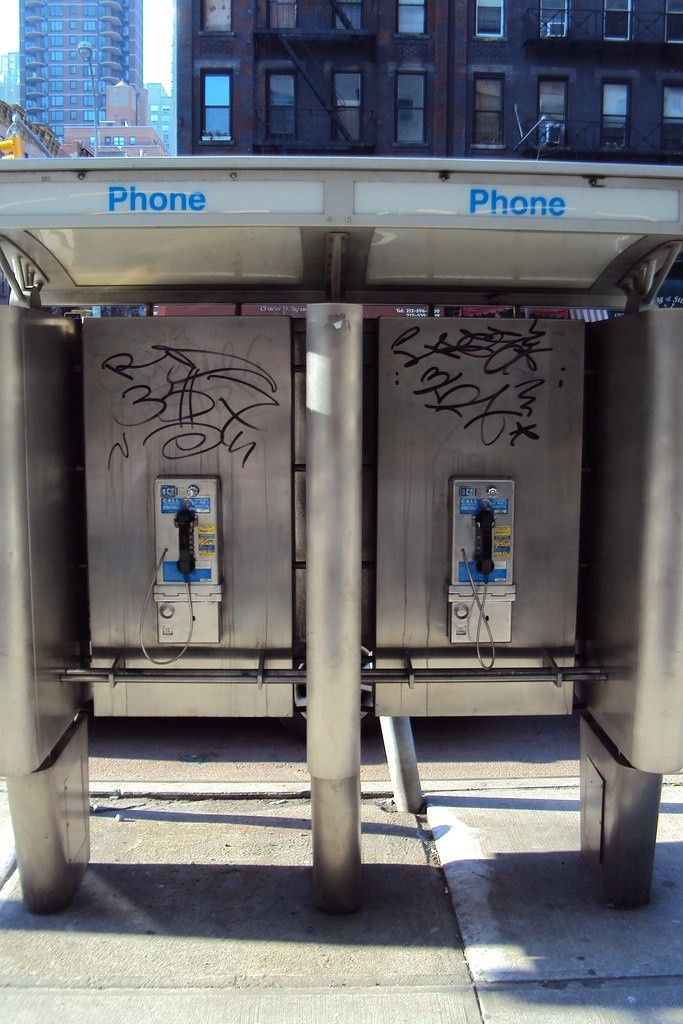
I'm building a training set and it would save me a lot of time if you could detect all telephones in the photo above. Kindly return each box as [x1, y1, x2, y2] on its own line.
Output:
[173, 509, 197, 574]
[472, 506, 496, 575]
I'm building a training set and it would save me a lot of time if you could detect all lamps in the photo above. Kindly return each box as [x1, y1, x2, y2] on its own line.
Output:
[77, 41, 94, 59]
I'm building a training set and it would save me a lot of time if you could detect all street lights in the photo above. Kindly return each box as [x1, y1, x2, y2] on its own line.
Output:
[78, 40, 100, 157]
